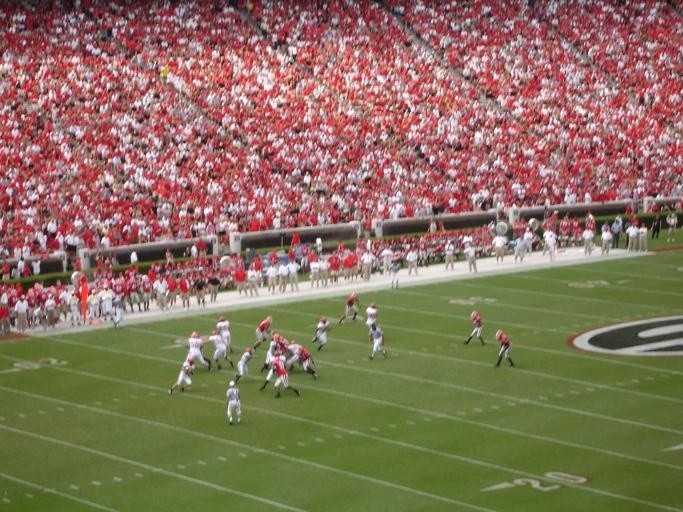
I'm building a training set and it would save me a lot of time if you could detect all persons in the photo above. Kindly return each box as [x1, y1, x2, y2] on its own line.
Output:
[253, 316, 273, 352]
[260, 333, 317, 398]
[235, 348, 253, 383]
[312, 315, 329, 350]
[169, 357, 195, 394]
[465, 311, 485, 344]
[0, 0, 682, 334]
[216, 316, 232, 354]
[226, 381, 242, 425]
[208, 330, 234, 370]
[187, 331, 210, 369]
[368, 324, 387, 360]
[495, 329, 515, 368]
